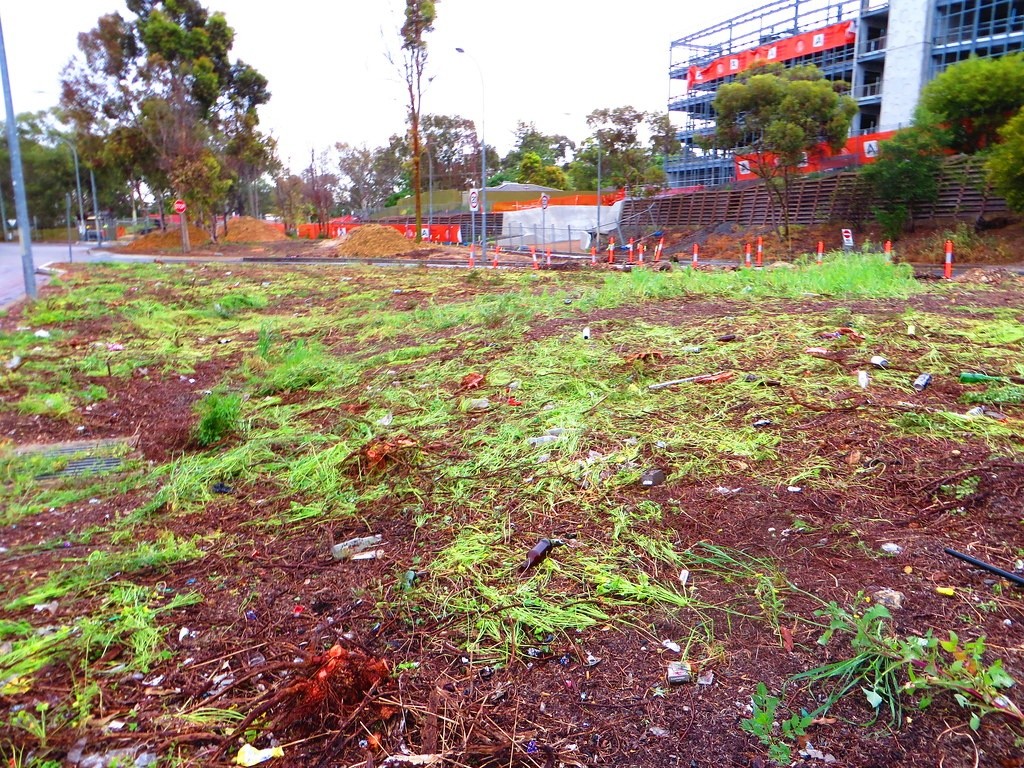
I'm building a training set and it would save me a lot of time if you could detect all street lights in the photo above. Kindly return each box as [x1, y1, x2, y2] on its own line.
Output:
[30, 134, 88, 242]
[453, 46, 487, 266]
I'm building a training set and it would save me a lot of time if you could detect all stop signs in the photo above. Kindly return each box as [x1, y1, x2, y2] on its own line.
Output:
[174, 200, 186, 213]
[844, 230, 851, 238]
